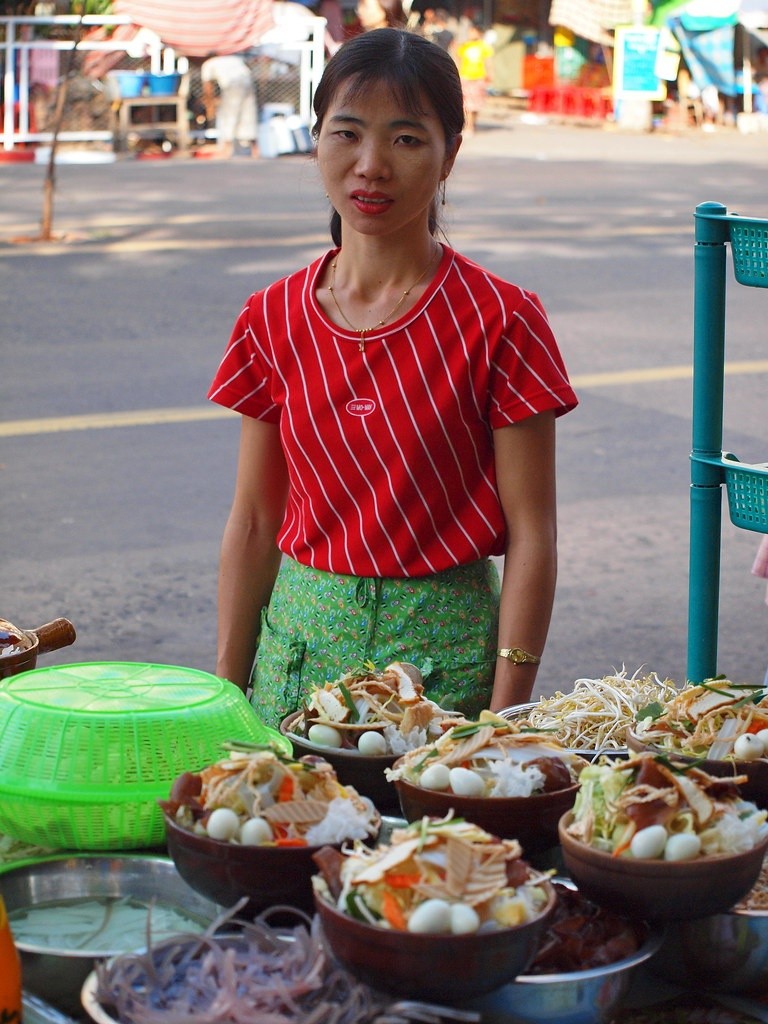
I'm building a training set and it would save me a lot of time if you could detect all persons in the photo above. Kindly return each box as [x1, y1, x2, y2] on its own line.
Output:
[419, 7, 495, 137]
[207, 28, 579, 731]
[201, 52, 260, 159]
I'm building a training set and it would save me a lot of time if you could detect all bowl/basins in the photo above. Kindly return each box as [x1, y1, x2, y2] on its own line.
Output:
[0, 685, 768, 1024]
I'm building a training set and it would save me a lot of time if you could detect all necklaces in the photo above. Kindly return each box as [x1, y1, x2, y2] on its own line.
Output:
[328, 241, 438, 351]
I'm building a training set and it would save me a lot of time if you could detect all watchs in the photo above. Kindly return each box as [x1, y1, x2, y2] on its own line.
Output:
[497, 647, 541, 665]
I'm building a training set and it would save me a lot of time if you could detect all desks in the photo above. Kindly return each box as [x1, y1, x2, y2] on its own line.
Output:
[119, 97, 187, 152]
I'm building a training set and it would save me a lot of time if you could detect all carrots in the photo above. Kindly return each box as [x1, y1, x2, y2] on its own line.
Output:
[381, 868, 447, 932]
[268, 776, 308, 846]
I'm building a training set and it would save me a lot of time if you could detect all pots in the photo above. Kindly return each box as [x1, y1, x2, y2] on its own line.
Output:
[0, 617, 77, 680]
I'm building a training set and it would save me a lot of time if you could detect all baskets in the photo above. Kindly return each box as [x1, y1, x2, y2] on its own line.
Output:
[0, 662, 294, 851]
